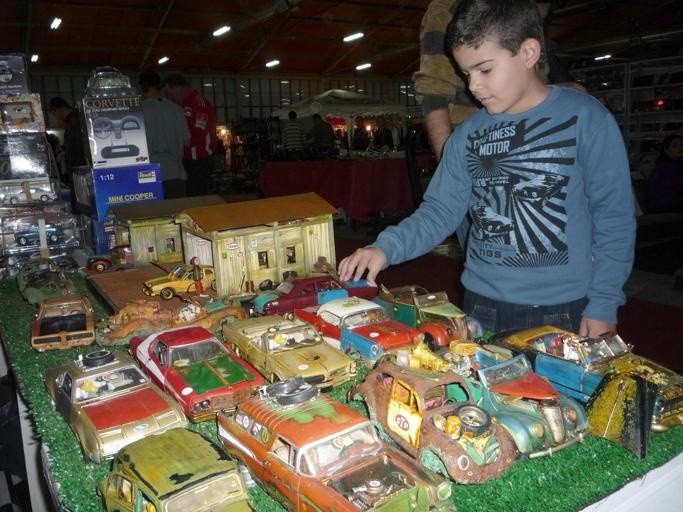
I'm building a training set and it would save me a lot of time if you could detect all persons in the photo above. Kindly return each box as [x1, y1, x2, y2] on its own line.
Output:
[161, 72, 217, 197]
[47, 96, 86, 171]
[307, 113, 335, 161]
[137, 68, 190, 200]
[372, 114, 394, 148]
[337, 0, 638, 348]
[282, 111, 307, 162]
[411, 1, 586, 254]
[543, 13, 577, 84]
[220, 139, 227, 166]
[349, 116, 370, 150]
[639, 134, 683, 291]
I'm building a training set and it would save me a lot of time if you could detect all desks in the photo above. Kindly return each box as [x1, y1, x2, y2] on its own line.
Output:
[0, 273, 683, 510]
